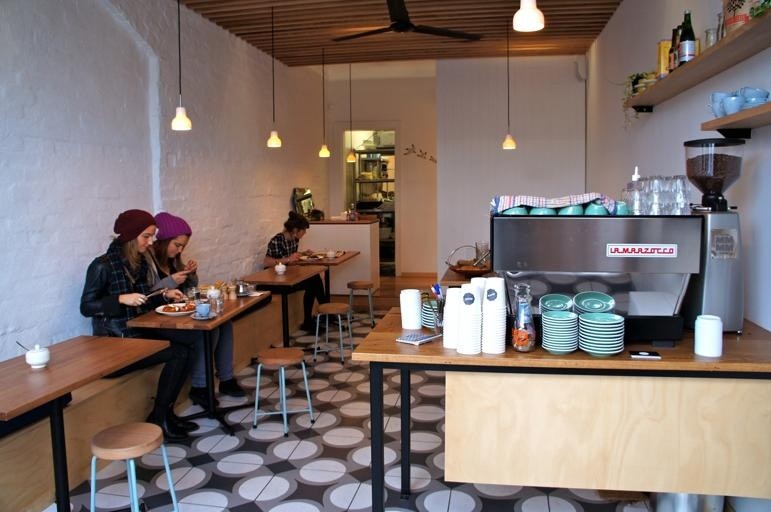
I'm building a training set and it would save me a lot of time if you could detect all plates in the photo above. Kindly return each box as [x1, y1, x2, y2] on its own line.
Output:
[524, 278, 552, 304]
[570, 278, 613, 298]
[537, 290, 626, 358]
[299, 255, 323, 261]
[154, 303, 194, 317]
[190, 311, 216, 320]
[421, 299, 438, 332]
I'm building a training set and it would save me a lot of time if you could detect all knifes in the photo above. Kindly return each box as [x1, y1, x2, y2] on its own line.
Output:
[144, 289, 167, 298]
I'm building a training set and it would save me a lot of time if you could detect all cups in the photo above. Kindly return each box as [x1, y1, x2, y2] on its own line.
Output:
[475, 241, 488, 262]
[704, 28, 717, 47]
[185, 287, 201, 304]
[623, 175, 689, 216]
[213, 294, 224, 313]
[441, 276, 506, 356]
[637, 72, 656, 94]
[707, 86, 771, 120]
[196, 303, 211, 316]
[246, 284, 255, 295]
[399, 288, 422, 332]
[325, 248, 335, 258]
[692, 313, 723, 358]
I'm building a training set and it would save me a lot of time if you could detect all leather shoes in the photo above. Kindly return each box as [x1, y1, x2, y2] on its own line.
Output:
[147, 399, 199, 441]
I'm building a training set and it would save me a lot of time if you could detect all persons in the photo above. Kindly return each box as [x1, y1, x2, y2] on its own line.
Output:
[79, 208, 200, 440]
[153, 211, 245, 406]
[261, 210, 336, 331]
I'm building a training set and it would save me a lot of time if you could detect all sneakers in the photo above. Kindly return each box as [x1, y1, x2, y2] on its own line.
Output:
[219, 378, 246, 396]
[189, 386, 220, 407]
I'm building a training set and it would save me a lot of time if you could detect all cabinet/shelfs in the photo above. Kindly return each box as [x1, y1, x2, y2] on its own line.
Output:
[352, 148, 395, 266]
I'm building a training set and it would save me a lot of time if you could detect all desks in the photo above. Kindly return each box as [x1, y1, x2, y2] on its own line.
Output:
[352, 305, 771, 511]
[299, 217, 381, 296]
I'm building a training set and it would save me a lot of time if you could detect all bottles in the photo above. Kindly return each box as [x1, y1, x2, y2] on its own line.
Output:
[715, 11, 726, 42]
[206, 286, 220, 300]
[668, 8, 697, 74]
[371, 192, 383, 202]
[511, 294, 536, 353]
[365, 162, 372, 172]
[273, 262, 286, 275]
[511, 282, 534, 316]
[220, 283, 243, 300]
[348, 208, 356, 220]
[23, 344, 50, 369]
[630, 164, 640, 182]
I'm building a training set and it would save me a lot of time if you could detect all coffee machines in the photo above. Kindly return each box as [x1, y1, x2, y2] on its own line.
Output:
[682, 136, 750, 335]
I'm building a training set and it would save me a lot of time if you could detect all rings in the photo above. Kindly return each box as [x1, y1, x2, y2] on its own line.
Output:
[138, 297, 144, 301]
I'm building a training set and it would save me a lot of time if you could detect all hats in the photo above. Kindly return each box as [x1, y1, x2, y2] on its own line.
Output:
[113, 210, 156, 244]
[153, 212, 192, 240]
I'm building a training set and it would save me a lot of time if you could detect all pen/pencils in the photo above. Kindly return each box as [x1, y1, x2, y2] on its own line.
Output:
[429, 283, 445, 313]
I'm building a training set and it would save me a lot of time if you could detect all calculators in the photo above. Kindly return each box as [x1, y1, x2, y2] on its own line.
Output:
[395, 332, 442, 346]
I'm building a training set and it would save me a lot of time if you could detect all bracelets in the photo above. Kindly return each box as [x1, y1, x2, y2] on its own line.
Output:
[163, 292, 169, 302]
[288, 257, 290, 263]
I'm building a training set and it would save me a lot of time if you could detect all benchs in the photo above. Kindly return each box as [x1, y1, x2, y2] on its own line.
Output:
[0, 290, 303, 511]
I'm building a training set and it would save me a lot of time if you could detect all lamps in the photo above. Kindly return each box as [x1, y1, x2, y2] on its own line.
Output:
[346, 62, 356, 163]
[170, 0, 191, 131]
[318, 47, 330, 157]
[266, 6, 282, 148]
[502, 17, 516, 150]
[512, 0, 544, 32]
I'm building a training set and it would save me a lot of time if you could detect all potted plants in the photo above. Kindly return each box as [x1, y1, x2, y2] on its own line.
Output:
[620, 72, 654, 135]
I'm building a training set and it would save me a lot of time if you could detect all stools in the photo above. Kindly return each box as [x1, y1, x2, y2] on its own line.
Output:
[253, 346, 315, 437]
[89, 422, 179, 511]
[347, 280, 376, 331]
[313, 302, 354, 365]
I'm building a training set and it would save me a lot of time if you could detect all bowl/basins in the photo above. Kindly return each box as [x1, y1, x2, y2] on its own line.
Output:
[502, 200, 626, 215]
[378, 227, 391, 240]
[363, 144, 376, 149]
[360, 172, 372, 180]
[356, 201, 382, 209]
